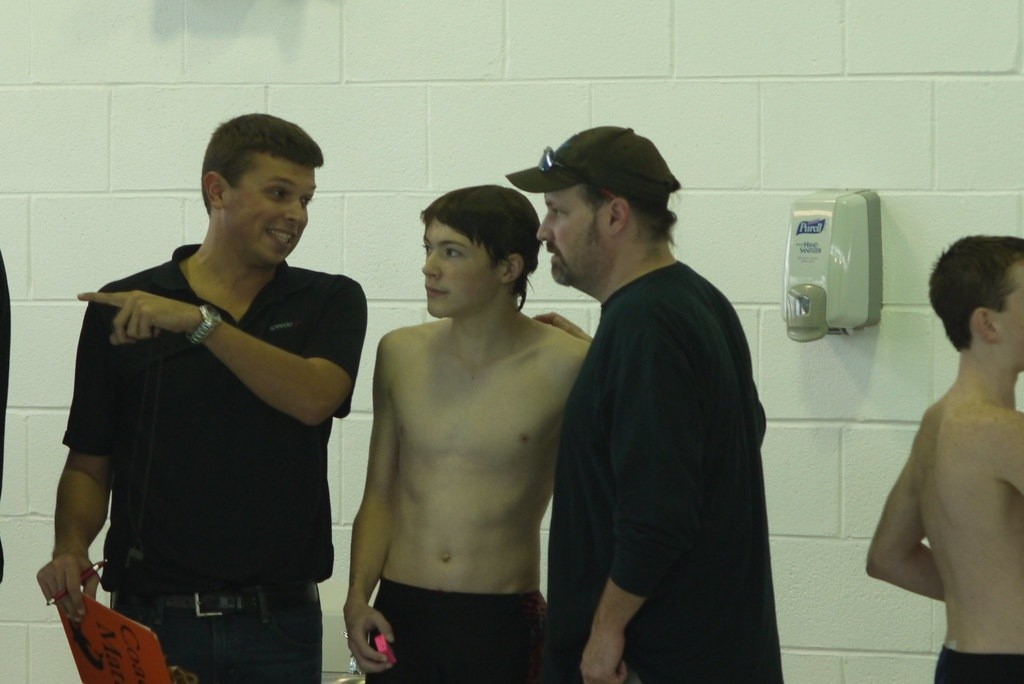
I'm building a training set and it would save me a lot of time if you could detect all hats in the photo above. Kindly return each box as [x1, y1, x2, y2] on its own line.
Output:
[506, 126, 671, 205]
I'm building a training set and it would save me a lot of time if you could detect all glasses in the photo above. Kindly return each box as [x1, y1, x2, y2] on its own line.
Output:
[537, 146, 618, 200]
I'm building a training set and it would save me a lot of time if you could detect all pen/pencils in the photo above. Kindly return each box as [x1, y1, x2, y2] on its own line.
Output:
[48, 559, 107, 605]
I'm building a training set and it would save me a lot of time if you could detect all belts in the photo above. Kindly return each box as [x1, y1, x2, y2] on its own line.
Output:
[113, 583, 319, 617]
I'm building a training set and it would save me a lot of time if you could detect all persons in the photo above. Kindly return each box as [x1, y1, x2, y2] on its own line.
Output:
[507, 126, 784, 684]
[36, 114, 367, 684]
[343, 185, 591, 684]
[866, 235, 1024, 684]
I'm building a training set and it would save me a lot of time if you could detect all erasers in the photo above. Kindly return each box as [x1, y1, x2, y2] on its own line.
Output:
[374, 635, 396, 665]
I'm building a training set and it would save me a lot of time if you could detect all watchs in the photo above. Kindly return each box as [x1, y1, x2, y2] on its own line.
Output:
[186, 305, 221, 344]
